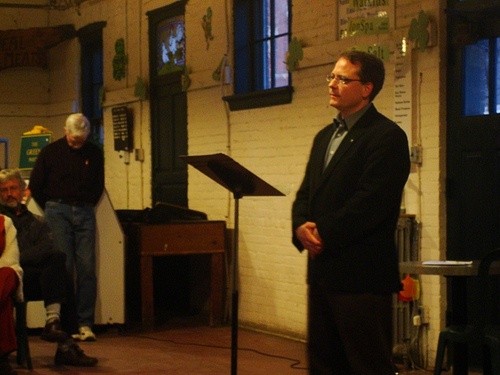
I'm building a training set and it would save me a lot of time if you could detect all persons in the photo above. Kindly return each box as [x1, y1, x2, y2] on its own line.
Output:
[0, 213, 23, 375]
[0, 168, 98, 366]
[29, 113, 104, 340]
[292, 50, 411, 375]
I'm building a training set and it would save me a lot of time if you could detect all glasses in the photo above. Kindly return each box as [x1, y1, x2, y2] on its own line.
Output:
[325, 72, 362, 86]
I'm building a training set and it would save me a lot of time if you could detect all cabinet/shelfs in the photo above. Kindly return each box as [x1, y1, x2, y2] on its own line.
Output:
[139, 220, 227, 332]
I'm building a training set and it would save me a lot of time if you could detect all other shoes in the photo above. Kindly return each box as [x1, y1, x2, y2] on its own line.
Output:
[55, 344, 98, 366]
[78, 326, 96, 341]
[41, 321, 71, 343]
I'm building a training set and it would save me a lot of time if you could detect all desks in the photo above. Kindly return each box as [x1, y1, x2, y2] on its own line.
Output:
[398, 260, 480, 276]
